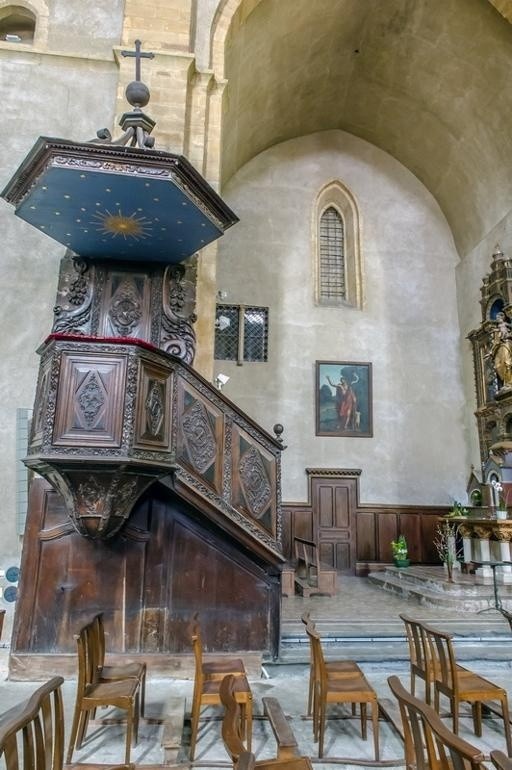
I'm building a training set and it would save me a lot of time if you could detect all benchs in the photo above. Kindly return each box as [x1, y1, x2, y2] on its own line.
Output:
[294, 536, 338, 595]
[161, 697, 186, 764]
[376, 698, 426, 750]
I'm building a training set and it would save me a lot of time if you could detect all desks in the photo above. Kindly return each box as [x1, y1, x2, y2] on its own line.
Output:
[435, 515, 511, 576]
[471, 559, 512, 615]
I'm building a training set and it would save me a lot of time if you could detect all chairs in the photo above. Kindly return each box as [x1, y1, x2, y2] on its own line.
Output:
[301, 611, 381, 762]
[387, 675, 511, 770]
[1, 675, 67, 770]
[66, 612, 145, 763]
[188, 621, 253, 754]
[219, 672, 313, 770]
[400, 614, 511, 760]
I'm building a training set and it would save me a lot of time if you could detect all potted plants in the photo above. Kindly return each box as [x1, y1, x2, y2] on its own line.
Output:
[389, 533, 411, 568]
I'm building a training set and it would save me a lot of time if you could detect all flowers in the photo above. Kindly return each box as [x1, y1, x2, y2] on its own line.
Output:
[489, 480, 510, 510]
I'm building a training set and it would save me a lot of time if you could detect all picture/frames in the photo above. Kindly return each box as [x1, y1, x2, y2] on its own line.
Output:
[314, 359, 374, 438]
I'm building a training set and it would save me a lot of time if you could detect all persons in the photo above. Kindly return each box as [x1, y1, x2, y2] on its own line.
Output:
[482, 303, 512, 389]
[325, 372, 362, 430]
[490, 311, 512, 342]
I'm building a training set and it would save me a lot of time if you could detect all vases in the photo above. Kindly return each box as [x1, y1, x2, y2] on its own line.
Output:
[495, 509, 507, 520]
